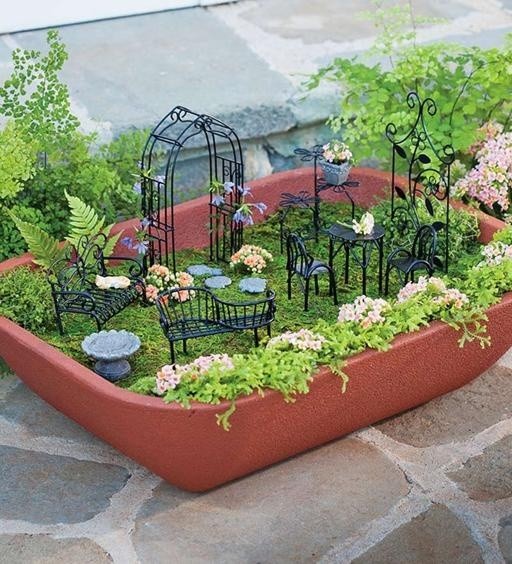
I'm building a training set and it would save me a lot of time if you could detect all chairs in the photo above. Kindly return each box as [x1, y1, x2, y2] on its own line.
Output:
[384, 224, 437, 295]
[286, 234, 338, 311]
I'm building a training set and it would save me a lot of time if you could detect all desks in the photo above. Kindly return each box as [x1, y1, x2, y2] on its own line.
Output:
[329, 222, 386, 298]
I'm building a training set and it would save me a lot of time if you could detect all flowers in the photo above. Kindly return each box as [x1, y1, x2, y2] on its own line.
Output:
[322, 138, 353, 162]
[229, 244, 274, 275]
[336, 211, 375, 235]
[140, 263, 197, 303]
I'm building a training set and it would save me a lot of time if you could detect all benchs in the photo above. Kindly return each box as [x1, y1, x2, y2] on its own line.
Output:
[156, 286, 275, 367]
[46, 234, 147, 336]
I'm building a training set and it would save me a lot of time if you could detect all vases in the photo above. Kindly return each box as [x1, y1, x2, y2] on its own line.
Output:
[320, 160, 352, 186]
